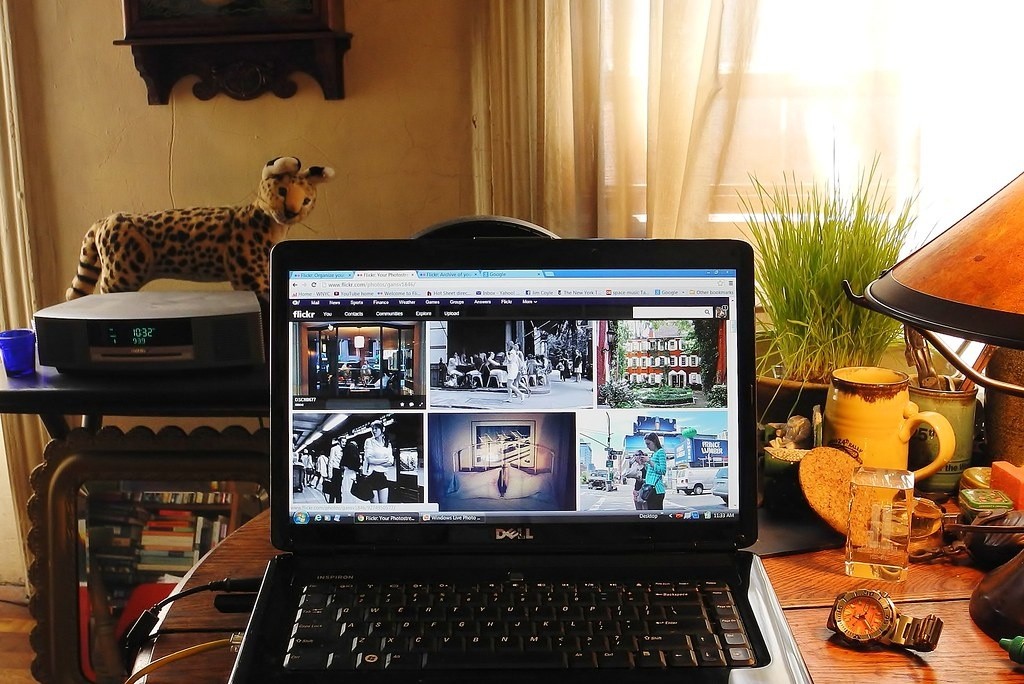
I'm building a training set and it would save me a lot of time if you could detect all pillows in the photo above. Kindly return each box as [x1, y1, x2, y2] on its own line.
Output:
[446, 468, 503, 500]
[502, 463, 555, 504]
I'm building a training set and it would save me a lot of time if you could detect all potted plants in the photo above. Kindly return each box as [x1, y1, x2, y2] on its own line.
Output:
[733, 143, 936, 458]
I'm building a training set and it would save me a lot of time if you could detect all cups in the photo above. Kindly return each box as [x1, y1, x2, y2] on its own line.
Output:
[0, 330, 36, 378]
[910, 376, 984, 489]
[845, 467, 915, 583]
[823, 367, 956, 483]
[759, 446, 810, 502]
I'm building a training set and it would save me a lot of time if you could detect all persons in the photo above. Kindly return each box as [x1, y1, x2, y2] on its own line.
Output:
[299, 419, 394, 503]
[631, 432, 668, 511]
[446, 339, 586, 402]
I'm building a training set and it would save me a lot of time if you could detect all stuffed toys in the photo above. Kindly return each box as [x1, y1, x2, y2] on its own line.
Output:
[64, 153, 335, 299]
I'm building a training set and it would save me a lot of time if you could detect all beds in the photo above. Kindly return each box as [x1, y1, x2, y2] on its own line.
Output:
[439, 440, 561, 513]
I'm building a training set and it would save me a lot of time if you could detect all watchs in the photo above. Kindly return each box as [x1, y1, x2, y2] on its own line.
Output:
[822, 586, 945, 657]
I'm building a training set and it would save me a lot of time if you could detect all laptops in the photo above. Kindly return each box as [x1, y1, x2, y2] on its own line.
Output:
[229, 238, 814, 684]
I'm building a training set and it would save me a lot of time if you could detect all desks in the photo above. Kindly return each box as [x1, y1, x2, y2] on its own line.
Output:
[2, 362, 271, 450]
[127, 507, 1024, 683]
[456, 363, 476, 386]
[490, 365, 507, 373]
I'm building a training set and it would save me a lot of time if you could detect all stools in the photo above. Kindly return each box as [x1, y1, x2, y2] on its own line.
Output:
[445, 369, 549, 388]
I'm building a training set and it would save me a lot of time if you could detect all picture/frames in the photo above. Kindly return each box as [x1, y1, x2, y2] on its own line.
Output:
[26, 425, 271, 684]
